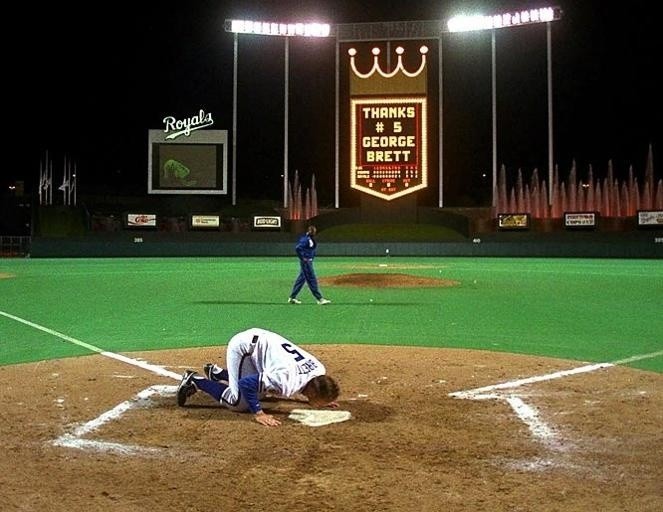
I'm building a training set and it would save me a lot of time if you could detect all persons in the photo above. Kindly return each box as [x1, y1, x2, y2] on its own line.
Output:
[176, 328, 340, 428]
[288, 225, 331, 306]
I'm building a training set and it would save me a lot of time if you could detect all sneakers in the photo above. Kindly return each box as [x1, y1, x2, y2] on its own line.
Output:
[203, 362, 223, 384]
[177, 369, 205, 407]
[316, 298, 331, 305]
[288, 298, 301, 304]
